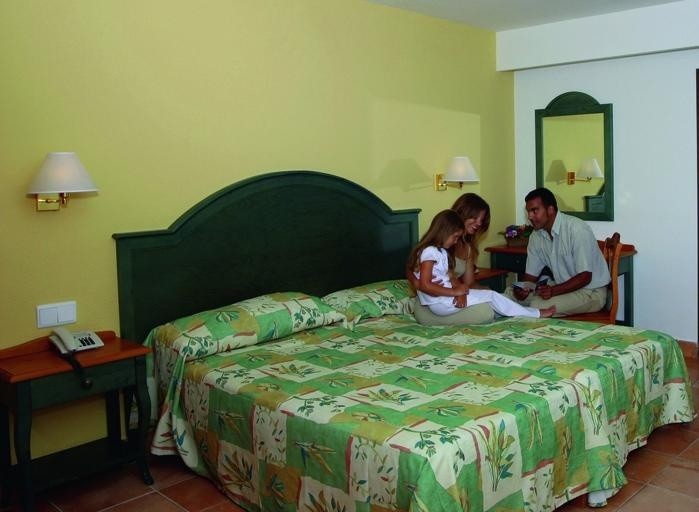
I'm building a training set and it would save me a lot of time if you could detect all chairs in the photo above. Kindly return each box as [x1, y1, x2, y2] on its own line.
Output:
[553, 232, 623, 324]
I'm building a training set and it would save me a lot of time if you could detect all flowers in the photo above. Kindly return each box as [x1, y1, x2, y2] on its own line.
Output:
[496, 223, 535, 239]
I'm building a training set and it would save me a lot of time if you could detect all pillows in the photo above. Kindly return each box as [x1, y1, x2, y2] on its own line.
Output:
[320, 279, 420, 331]
[141, 291, 347, 362]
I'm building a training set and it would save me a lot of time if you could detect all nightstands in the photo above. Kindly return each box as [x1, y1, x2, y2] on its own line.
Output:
[457, 265, 509, 294]
[0, 330, 154, 511]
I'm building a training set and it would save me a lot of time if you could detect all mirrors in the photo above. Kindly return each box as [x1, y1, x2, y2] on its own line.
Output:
[533, 90, 614, 222]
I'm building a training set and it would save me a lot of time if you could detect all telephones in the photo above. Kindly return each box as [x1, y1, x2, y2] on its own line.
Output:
[48, 327, 104, 375]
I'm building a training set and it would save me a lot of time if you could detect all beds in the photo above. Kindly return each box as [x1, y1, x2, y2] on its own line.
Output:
[109, 169, 697, 510]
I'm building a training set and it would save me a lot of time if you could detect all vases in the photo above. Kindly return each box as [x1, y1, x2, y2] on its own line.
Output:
[504, 236, 529, 247]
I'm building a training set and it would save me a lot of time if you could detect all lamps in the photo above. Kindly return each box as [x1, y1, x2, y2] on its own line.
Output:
[434, 155, 480, 193]
[566, 157, 604, 186]
[24, 151, 101, 212]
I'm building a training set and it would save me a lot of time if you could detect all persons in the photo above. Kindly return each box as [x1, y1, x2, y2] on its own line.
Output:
[501, 187, 612, 318]
[404, 192, 503, 325]
[406, 208, 557, 320]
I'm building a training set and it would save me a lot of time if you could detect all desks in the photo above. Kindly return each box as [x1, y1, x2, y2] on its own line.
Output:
[484, 240, 638, 327]
